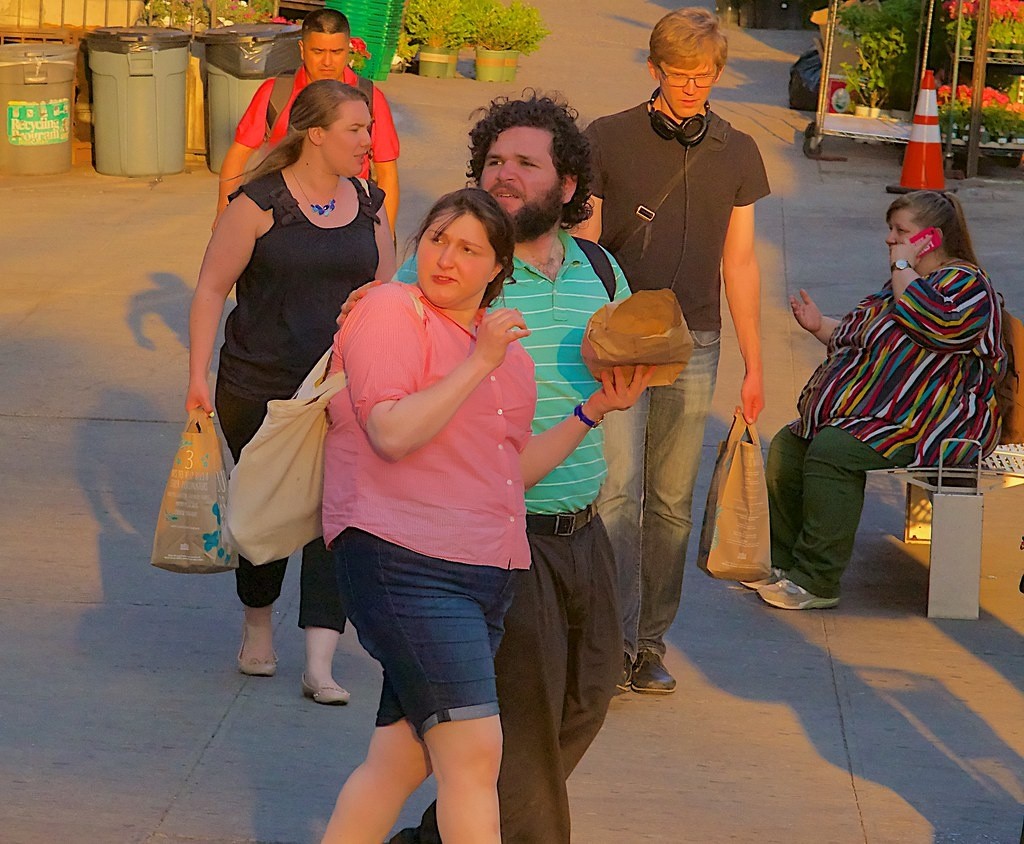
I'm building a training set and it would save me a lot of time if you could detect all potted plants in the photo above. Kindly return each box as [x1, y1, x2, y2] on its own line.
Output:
[406, 0, 553, 80]
[843, 2, 922, 119]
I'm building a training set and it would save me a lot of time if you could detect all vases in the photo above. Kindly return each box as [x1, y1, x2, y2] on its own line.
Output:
[940, 108, 1024, 144]
[947, 35, 1024, 59]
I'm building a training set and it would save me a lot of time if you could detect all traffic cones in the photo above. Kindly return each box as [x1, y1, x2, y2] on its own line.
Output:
[886, 69, 959, 195]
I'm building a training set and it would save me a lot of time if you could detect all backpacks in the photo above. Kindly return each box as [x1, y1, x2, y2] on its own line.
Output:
[955, 263, 1024, 445]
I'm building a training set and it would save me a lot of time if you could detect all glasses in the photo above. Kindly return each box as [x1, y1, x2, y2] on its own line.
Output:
[657, 64, 719, 87]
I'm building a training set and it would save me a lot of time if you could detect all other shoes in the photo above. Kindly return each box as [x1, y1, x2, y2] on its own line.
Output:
[302, 670, 350, 704]
[238, 625, 278, 675]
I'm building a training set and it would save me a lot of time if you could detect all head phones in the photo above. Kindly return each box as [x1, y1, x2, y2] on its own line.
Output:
[647, 86, 711, 146]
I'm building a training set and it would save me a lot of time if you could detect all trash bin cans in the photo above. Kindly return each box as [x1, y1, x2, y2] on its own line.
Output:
[0, 43, 77, 175]
[74, 39, 94, 142]
[87, 26, 190, 178]
[185, 40, 208, 156]
[203, 22, 303, 173]
[810, 0, 861, 77]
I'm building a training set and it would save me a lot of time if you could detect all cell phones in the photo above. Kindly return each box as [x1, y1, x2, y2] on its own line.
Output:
[909, 227, 941, 258]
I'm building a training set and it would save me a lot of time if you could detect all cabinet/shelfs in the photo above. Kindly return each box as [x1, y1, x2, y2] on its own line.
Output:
[933, 0, 1024, 178]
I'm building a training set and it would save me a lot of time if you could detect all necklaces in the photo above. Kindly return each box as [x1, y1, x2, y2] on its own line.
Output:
[288, 164, 339, 217]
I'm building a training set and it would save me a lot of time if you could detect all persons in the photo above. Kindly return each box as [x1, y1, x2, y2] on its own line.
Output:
[317, 187, 657, 844]
[187, 79, 397, 706]
[337, 86, 635, 844]
[739, 189, 1010, 610]
[562, 6, 771, 696]
[208, 7, 400, 245]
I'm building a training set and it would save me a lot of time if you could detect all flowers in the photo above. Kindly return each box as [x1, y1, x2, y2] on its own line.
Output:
[942, 0, 1022, 43]
[937, 81, 1024, 119]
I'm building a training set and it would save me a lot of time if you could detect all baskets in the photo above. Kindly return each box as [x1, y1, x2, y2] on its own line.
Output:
[324, 1, 406, 81]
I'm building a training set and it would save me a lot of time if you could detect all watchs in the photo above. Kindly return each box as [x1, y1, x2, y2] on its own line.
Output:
[573, 400, 603, 429]
[887, 258, 915, 273]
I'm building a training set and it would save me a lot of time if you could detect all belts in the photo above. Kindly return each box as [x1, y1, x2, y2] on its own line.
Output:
[526, 504, 596, 535]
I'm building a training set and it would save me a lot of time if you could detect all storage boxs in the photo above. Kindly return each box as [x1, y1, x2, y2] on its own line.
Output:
[826, 75, 869, 112]
[323, 0, 405, 81]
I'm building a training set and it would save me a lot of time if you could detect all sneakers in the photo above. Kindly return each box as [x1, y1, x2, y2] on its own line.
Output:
[756, 579, 841, 609]
[615, 652, 633, 691]
[632, 651, 676, 694]
[739, 565, 786, 589]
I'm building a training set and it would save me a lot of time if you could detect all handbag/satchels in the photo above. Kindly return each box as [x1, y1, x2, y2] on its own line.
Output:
[697, 412, 771, 580]
[151, 409, 239, 574]
[226, 397, 323, 565]
